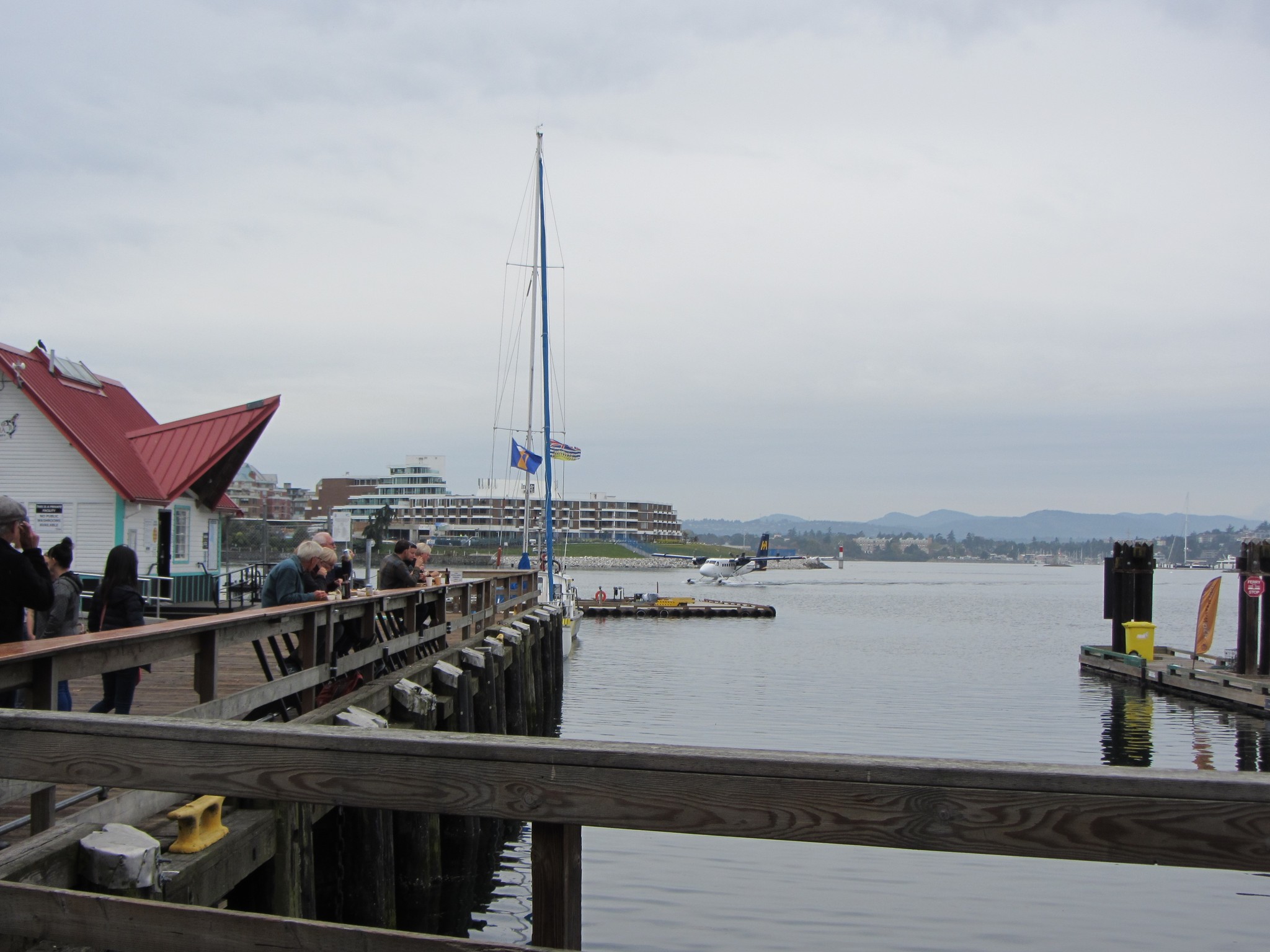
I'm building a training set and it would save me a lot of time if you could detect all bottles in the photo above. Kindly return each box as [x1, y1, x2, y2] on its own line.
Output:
[341, 575, 349, 600]
[346, 574, 351, 598]
[445, 568, 450, 584]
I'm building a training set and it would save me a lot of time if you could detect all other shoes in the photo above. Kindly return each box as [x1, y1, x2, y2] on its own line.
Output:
[276, 658, 298, 676]
[374, 664, 386, 678]
[358, 633, 378, 649]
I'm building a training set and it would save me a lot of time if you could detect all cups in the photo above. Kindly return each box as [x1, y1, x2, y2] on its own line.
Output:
[366, 585, 373, 596]
[425, 577, 432, 587]
[435, 577, 441, 586]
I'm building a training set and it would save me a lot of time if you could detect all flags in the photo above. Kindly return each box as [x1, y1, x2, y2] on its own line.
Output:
[548, 439, 581, 461]
[509, 438, 543, 474]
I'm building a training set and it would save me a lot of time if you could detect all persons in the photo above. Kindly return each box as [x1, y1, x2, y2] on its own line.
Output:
[259, 529, 370, 687]
[0, 494, 82, 712]
[376, 539, 441, 644]
[87, 546, 151, 715]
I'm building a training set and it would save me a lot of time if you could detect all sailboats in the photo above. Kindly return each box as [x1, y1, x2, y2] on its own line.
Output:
[1018, 546, 1106, 567]
[1153, 491, 1236, 570]
[446, 121, 585, 664]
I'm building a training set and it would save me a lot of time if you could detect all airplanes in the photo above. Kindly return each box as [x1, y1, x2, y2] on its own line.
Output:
[651, 532, 843, 587]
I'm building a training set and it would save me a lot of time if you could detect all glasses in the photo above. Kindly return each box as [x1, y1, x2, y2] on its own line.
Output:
[317, 558, 322, 562]
[329, 542, 334, 546]
[324, 561, 334, 567]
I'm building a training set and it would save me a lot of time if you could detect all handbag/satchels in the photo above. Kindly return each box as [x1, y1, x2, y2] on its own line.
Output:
[315, 669, 366, 707]
